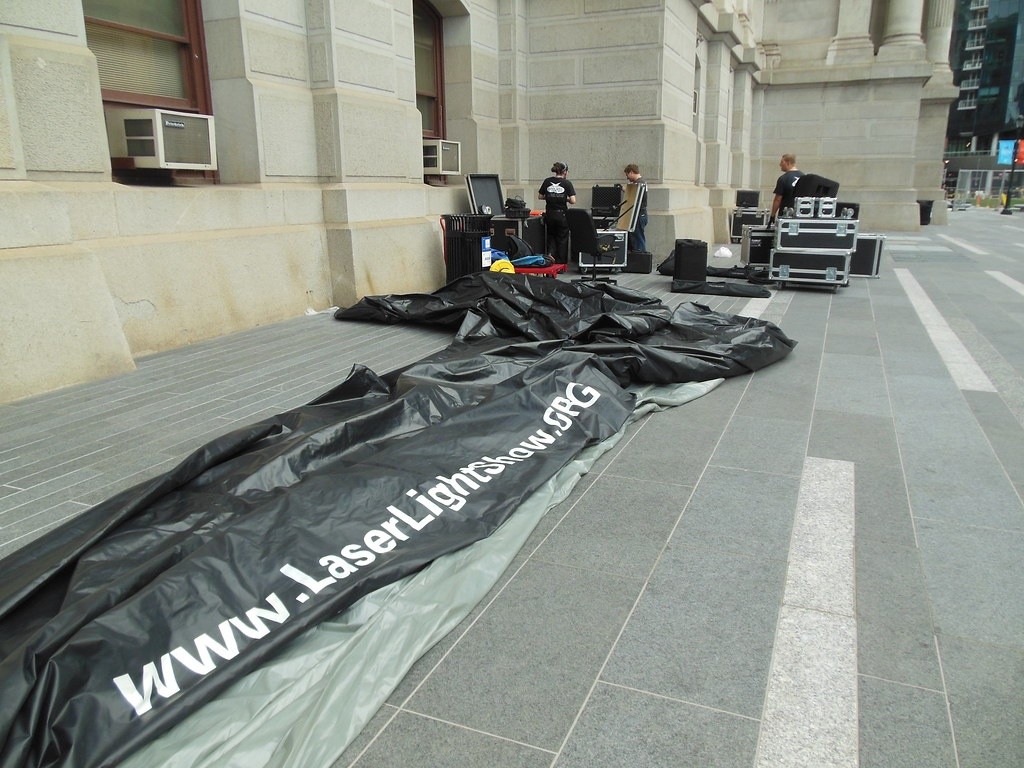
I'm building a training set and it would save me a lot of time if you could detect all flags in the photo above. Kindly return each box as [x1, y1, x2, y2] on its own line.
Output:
[997, 140, 1016, 165]
[1015, 139, 1024, 166]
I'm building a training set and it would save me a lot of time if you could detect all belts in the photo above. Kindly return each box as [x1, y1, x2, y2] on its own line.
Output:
[546, 209, 564, 213]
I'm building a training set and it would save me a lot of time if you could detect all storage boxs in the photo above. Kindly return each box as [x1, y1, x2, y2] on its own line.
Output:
[463, 172, 546, 256]
[732, 197, 887, 294]
[577, 182, 646, 275]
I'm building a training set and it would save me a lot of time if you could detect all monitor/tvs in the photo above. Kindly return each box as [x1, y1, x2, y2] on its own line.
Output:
[736, 190, 759, 208]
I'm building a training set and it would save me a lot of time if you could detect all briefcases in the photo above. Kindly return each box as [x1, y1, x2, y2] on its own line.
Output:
[621, 247, 652, 274]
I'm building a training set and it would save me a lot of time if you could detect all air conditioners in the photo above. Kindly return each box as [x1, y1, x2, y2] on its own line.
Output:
[108, 108, 219, 172]
[423, 138, 463, 176]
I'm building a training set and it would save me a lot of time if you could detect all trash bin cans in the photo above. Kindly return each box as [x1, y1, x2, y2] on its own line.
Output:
[440, 213, 494, 285]
[916, 200, 934, 225]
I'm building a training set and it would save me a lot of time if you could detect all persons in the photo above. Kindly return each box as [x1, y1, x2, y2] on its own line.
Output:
[538, 162, 576, 274]
[766, 153, 806, 230]
[620, 164, 649, 253]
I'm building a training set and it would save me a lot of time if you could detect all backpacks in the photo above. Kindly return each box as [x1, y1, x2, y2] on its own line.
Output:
[657, 249, 676, 275]
[505, 233, 535, 261]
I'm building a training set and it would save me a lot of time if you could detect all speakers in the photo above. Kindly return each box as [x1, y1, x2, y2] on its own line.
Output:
[793, 175, 840, 200]
[675, 239, 708, 281]
[591, 186, 622, 217]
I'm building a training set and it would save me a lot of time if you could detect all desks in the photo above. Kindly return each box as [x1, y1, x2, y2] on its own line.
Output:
[1014, 204, 1024, 212]
